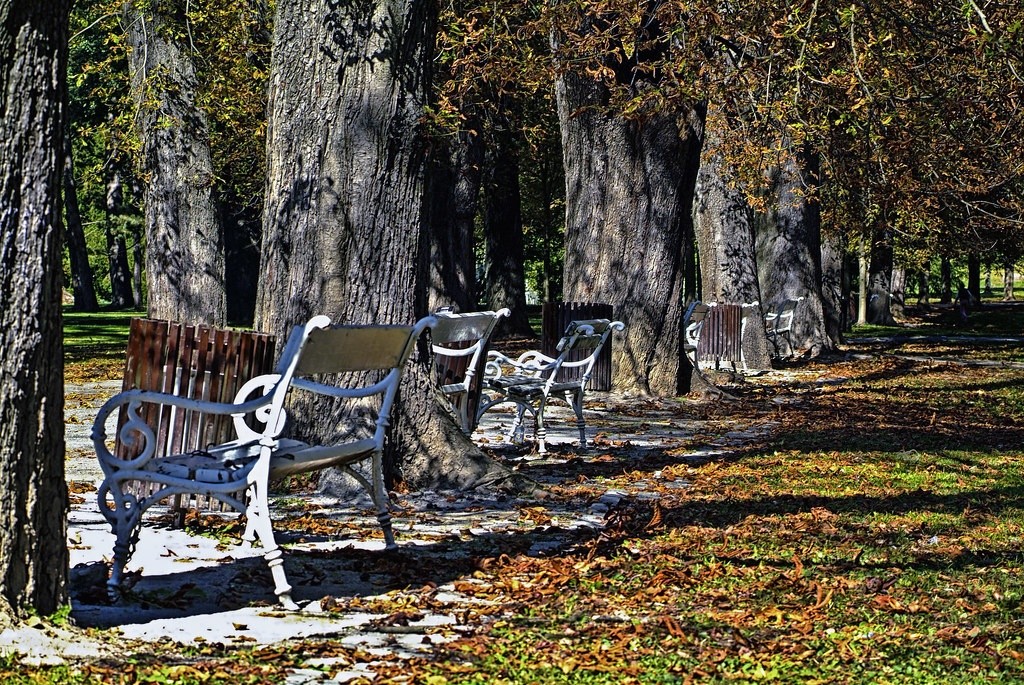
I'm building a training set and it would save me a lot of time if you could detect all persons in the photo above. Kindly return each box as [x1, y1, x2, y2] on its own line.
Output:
[953, 284, 977, 327]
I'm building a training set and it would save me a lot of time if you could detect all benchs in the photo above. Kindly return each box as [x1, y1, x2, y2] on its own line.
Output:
[90, 312, 439, 613]
[763, 296, 807, 359]
[715, 301, 759, 371]
[475, 315, 626, 463]
[683, 301, 718, 374]
[429, 305, 512, 440]
